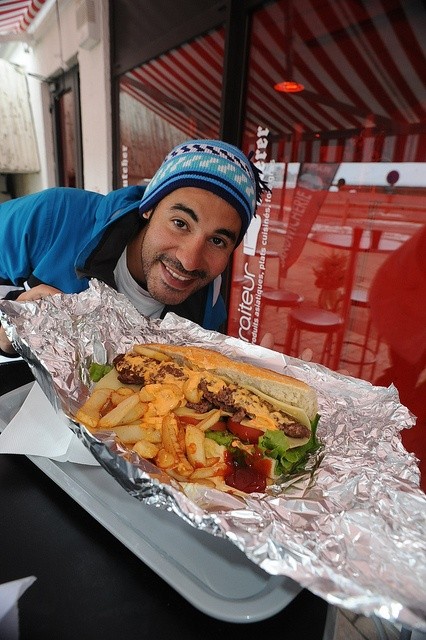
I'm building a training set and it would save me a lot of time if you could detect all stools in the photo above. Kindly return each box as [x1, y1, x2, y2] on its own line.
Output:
[319, 286, 379, 384]
[256, 287, 298, 349]
[243, 246, 281, 289]
[285, 299, 345, 366]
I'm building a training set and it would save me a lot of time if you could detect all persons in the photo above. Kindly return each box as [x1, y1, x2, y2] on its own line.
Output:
[0, 140, 258, 358]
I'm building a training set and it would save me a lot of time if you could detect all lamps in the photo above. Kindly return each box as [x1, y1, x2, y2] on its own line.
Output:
[274, 1, 306, 95]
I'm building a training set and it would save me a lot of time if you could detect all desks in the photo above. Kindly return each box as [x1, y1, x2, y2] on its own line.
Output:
[306, 232, 402, 371]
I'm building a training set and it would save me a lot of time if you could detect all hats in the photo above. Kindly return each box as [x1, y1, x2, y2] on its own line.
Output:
[139, 139, 257, 250]
